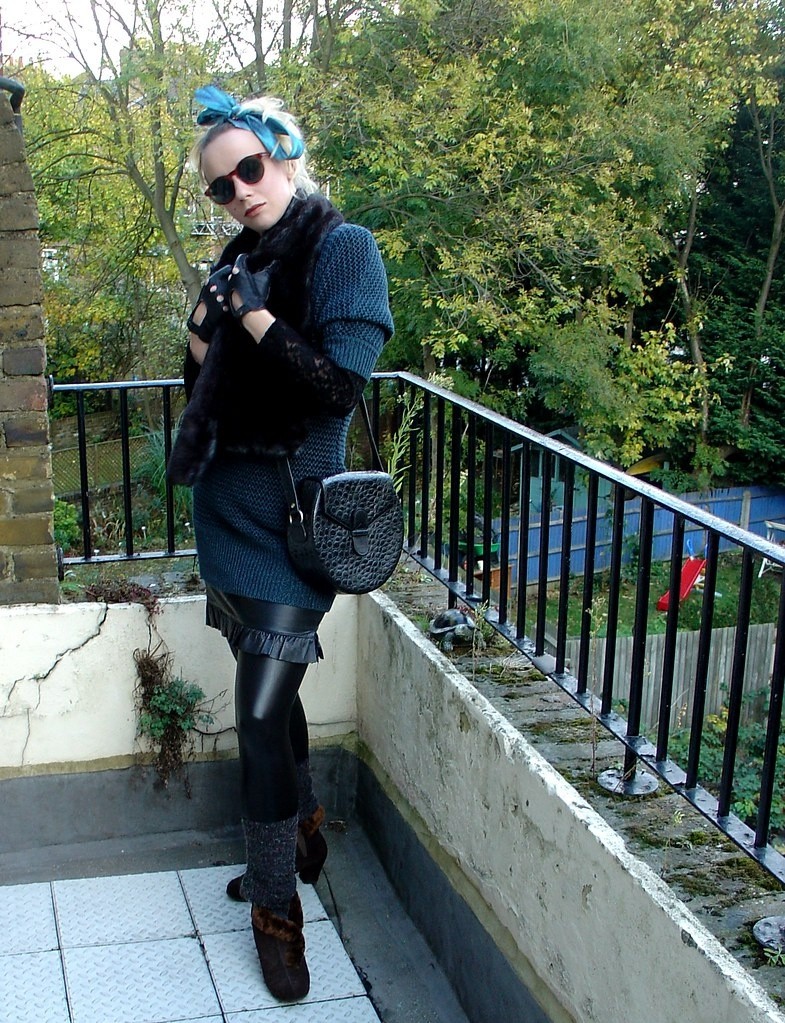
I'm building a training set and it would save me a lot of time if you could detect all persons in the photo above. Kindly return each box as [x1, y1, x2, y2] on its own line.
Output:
[168, 86, 393, 1005]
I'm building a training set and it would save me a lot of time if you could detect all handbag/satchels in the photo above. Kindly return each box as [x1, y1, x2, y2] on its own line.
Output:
[287, 470, 404, 594]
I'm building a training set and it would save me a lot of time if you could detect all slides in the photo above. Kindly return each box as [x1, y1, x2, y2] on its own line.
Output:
[656, 556, 708, 610]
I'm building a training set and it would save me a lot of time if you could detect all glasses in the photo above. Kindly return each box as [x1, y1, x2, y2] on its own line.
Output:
[203, 153, 270, 205]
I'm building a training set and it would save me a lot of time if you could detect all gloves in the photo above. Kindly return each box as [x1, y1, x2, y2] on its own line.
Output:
[187, 265, 233, 342]
[226, 254, 281, 319]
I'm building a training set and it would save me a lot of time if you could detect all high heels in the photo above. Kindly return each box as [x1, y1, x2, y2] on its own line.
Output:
[226, 807, 327, 902]
[250, 890, 310, 1003]
